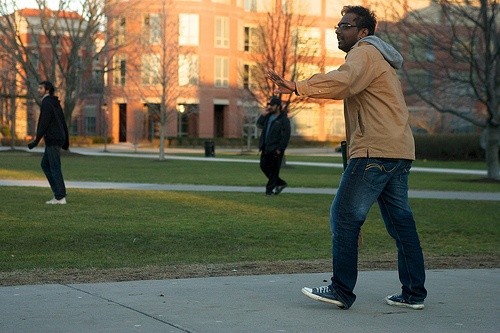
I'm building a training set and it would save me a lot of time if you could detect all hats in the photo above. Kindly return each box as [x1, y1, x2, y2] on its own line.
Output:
[267, 97, 281, 105]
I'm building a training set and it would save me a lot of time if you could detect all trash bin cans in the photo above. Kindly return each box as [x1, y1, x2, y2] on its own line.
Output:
[341, 140, 347, 171]
[204, 140, 215, 158]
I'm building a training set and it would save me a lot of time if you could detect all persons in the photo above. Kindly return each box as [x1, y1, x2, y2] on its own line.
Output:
[29, 80, 70, 204]
[266, 5, 427, 309]
[256, 98, 291, 196]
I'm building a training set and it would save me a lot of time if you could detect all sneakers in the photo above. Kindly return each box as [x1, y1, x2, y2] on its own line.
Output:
[46, 197, 66, 204]
[301, 285, 344, 307]
[385, 295, 424, 309]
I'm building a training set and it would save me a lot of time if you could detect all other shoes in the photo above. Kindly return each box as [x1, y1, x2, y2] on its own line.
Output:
[275, 185, 286, 194]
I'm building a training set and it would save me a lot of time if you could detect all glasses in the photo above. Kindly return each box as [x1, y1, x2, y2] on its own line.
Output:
[335, 24, 362, 30]
